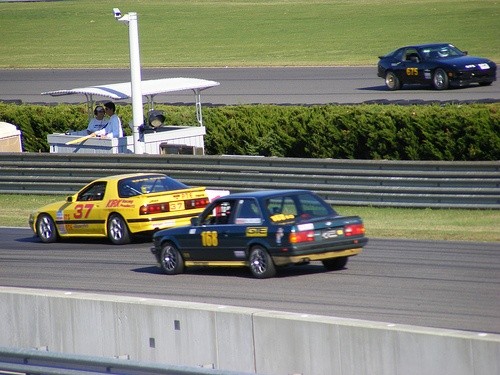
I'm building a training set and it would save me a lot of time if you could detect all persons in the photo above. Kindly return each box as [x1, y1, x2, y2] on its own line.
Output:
[87, 105, 110, 137]
[90, 102, 123, 138]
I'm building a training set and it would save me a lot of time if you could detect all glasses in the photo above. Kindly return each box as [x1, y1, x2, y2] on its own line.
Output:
[105, 108, 110, 111]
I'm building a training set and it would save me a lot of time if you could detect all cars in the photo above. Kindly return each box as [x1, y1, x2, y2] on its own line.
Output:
[150, 189, 368, 278]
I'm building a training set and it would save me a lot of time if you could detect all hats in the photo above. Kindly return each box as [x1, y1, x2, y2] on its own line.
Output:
[95, 106, 104, 112]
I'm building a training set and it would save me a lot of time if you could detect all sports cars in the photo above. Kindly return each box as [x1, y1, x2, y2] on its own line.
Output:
[377, 43, 496, 90]
[29, 173, 210, 245]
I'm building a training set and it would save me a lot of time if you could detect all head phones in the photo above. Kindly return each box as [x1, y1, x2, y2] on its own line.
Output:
[94, 106, 105, 115]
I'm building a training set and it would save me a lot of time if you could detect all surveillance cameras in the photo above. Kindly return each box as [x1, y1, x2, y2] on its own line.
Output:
[112, 8, 122, 20]
